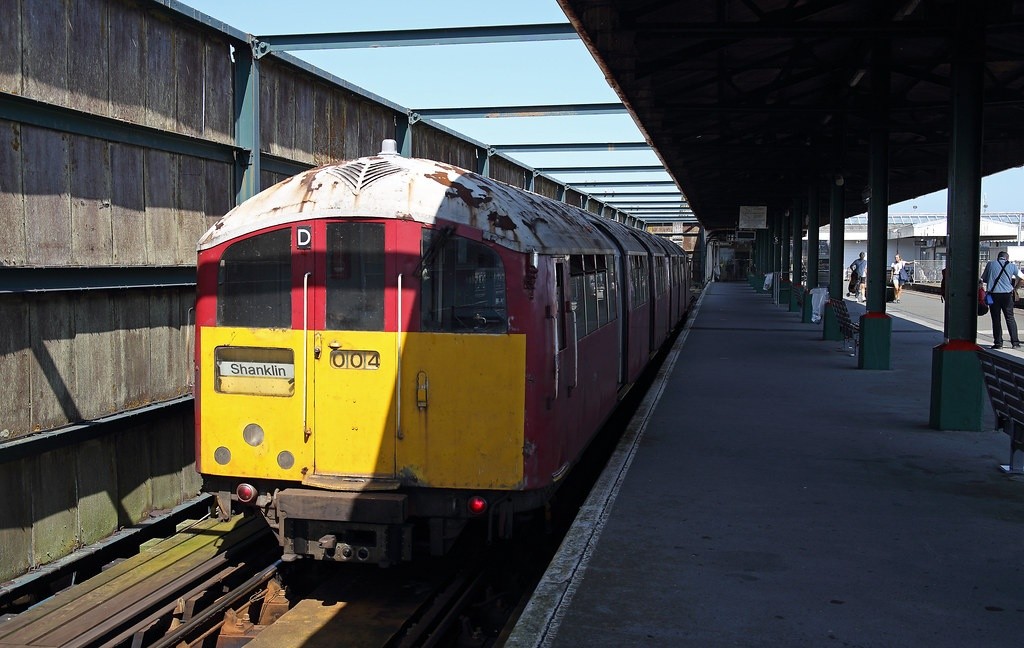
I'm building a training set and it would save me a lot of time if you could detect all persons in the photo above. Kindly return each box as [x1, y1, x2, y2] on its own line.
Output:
[889, 254, 906, 303]
[981, 251, 1024, 349]
[850, 252, 867, 303]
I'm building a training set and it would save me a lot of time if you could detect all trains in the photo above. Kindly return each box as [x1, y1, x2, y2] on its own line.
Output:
[185, 138, 694, 596]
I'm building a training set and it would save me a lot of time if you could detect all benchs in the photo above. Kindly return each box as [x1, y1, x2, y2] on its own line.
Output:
[977, 349, 1024, 474]
[830, 298, 860, 356]
[792, 284, 805, 317]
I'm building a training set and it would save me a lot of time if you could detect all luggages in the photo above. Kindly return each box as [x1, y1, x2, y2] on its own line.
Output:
[886, 280, 895, 302]
[847, 277, 860, 297]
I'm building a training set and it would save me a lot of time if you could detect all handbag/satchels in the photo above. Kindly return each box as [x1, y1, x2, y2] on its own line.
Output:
[851, 271, 859, 279]
[900, 263, 909, 282]
[978, 287, 988, 316]
[985, 291, 993, 305]
[1012, 289, 1019, 301]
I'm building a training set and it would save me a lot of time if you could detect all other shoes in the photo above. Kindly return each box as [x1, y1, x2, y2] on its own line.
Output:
[893, 300, 897, 303]
[1012, 345, 1021, 349]
[991, 344, 1003, 349]
[897, 299, 900, 303]
[862, 298, 866, 301]
